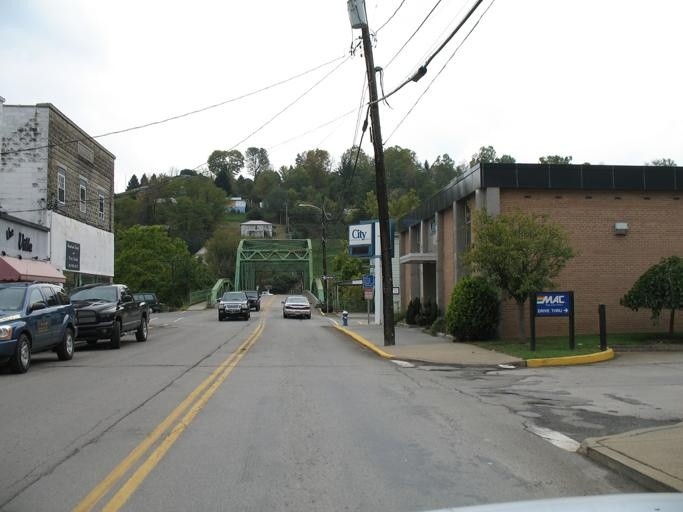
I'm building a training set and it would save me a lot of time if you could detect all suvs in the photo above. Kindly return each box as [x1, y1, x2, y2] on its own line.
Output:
[245, 290, 261, 311]
[217, 291, 250, 322]
[0, 282, 79, 373]
[134, 292, 159, 314]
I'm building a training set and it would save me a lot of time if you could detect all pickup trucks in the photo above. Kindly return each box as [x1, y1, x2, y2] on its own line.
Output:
[67, 283, 151, 349]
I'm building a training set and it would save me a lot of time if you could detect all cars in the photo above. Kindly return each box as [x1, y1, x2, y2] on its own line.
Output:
[281, 296, 312, 319]
